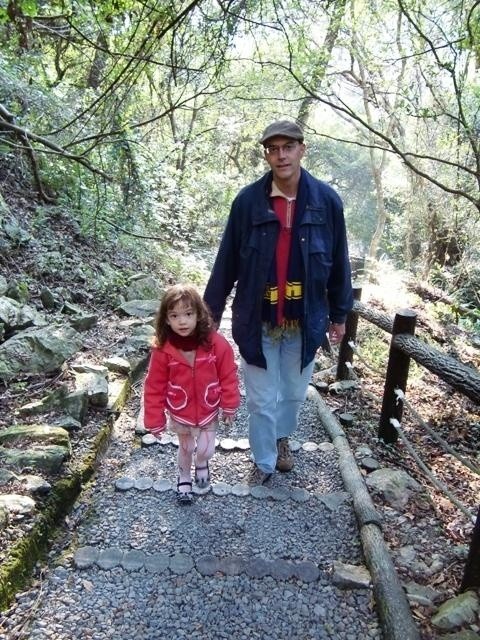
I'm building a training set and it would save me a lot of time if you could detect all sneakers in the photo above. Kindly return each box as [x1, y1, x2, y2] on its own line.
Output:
[176, 477, 195, 505]
[195, 460, 210, 489]
[276, 436, 294, 470]
[240, 463, 272, 488]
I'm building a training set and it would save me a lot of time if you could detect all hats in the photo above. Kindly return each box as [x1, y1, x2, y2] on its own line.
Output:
[259, 120, 304, 144]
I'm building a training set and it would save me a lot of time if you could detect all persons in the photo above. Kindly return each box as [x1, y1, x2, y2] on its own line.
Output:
[203, 122, 353, 487]
[144, 284, 239, 502]
